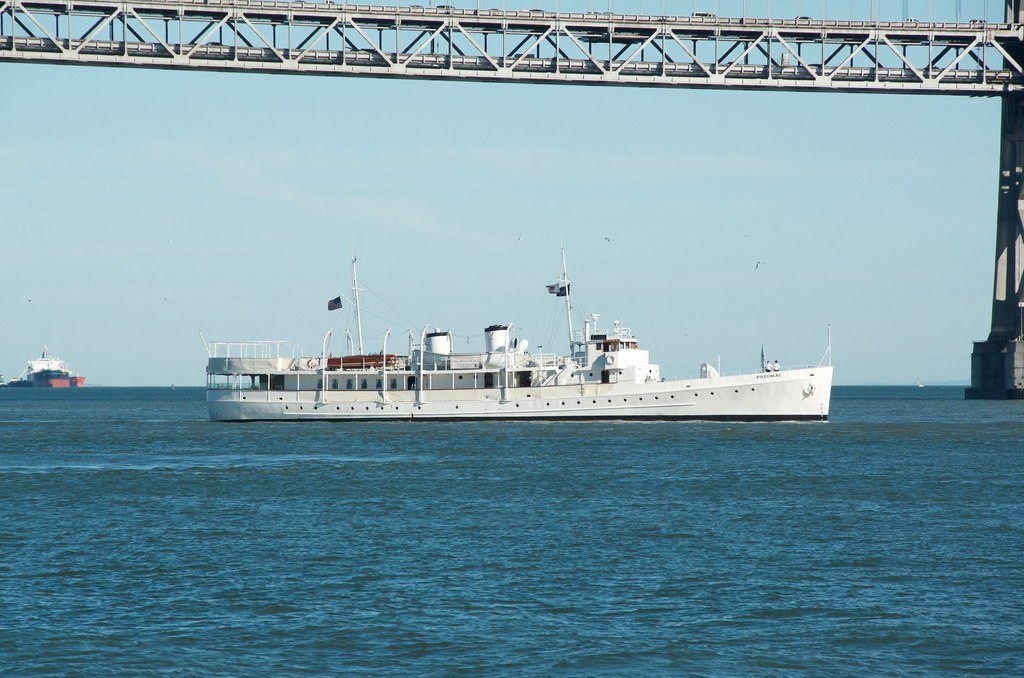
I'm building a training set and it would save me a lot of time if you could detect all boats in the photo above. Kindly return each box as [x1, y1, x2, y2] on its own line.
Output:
[203, 238, 835, 425]
[0, 347, 86, 388]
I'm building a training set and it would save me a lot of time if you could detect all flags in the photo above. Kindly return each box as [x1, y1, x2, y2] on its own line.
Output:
[546, 283, 560, 294]
[328, 297, 342, 310]
[556, 284, 570, 296]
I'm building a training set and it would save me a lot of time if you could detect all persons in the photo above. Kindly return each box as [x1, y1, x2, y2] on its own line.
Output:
[661, 378, 666, 382]
[774, 360, 780, 372]
[765, 361, 773, 372]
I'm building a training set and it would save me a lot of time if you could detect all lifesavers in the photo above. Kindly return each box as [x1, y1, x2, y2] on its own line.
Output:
[307, 359, 317, 369]
[605, 354, 615, 364]
